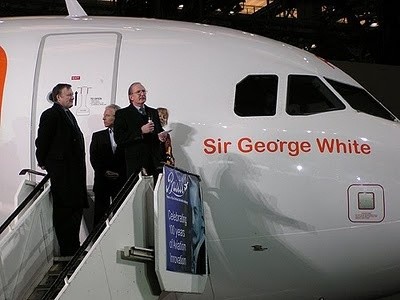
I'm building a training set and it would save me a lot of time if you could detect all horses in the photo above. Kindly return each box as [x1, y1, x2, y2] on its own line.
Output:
[158, 129, 173, 139]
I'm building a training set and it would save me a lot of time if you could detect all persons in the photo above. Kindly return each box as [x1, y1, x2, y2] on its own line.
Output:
[156, 108, 175, 167]
[89, 104, 126, 239]
[35, 84, 87, 255]
[114, 82, 166, 188]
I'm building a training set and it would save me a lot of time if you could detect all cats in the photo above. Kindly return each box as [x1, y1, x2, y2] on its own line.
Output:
[0, 0, 400, 297]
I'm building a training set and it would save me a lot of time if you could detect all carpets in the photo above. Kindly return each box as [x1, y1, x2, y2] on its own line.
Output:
[140, 108, 147, 115]
[66, 108, 75, 128]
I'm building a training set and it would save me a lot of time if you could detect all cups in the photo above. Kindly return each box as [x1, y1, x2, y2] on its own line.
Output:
[132, 90, 146, 94]
